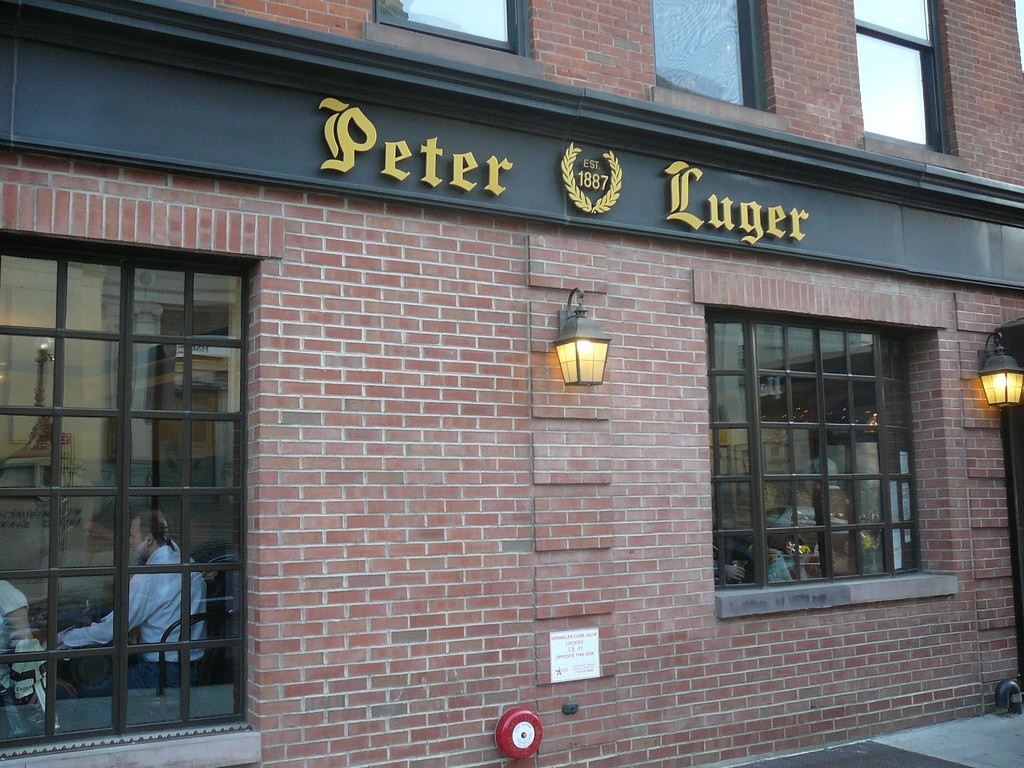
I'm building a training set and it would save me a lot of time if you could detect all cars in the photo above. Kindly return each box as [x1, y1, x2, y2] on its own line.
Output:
[81, 541, 241, 650]
[763, 505, 871, 560]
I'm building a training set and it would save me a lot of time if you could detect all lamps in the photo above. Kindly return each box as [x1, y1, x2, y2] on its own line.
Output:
[553, 286, 611, 387]
[976, 328, 1024, 406]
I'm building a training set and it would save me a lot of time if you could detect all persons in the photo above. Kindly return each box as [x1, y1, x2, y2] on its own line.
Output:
[0, 580, 78, 700]
[714, 558, 745, 581]
[60, 510, 207, 695]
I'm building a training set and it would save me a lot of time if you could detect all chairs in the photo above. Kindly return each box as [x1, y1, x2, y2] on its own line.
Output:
[158, 610, 238, 688]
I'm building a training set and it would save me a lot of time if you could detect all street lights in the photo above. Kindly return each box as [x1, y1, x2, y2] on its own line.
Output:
[979, 329, 1023, 699]
